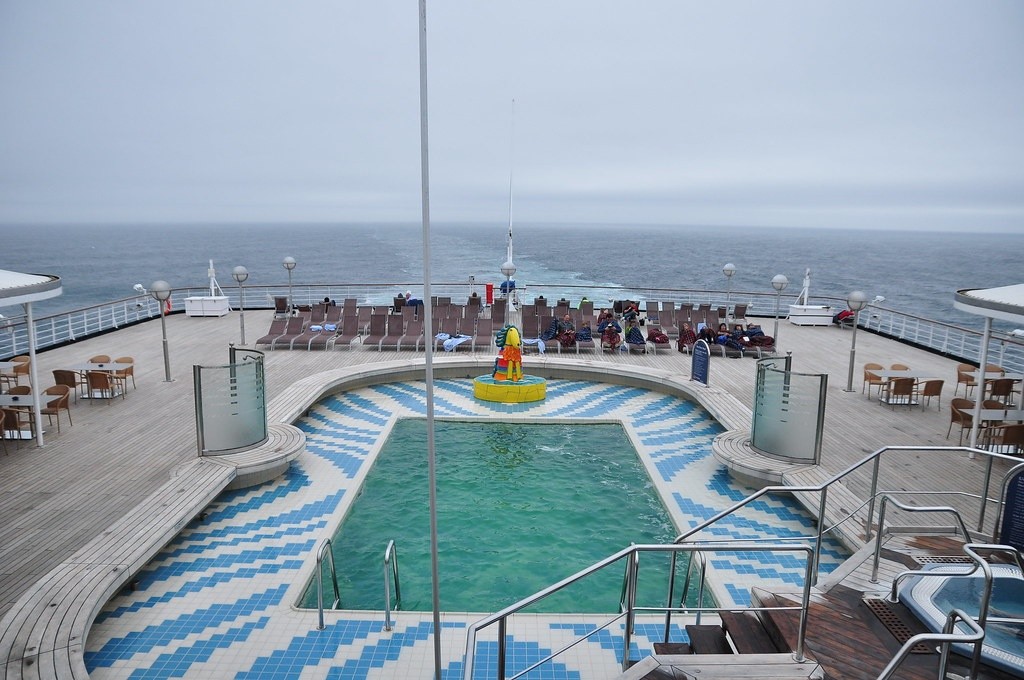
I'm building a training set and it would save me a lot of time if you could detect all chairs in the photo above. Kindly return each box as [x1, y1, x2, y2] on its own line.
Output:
[863, 362, 1024, 462]
[254, 295, 779, 364]
[0, 355, 138, 457]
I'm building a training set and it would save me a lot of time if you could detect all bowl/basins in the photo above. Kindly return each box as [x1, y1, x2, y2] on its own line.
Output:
[98, 364, 103, 367]
[12, 397, 19, 400]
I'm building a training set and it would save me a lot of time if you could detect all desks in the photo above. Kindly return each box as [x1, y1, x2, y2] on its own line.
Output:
[54, 363, 135, 399]
[0, 361, 27, 395]
[961, 371, 1024, 408]
[866, 369, 939, 405]
[0, 395, 64, 440]
[957, 409, 1024, 456]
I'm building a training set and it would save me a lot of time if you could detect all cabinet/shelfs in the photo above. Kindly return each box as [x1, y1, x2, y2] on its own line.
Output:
[184, 295, 230, 317]
[789, 304, 836, 327]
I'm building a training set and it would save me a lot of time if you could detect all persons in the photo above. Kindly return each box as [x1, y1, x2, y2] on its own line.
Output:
[702, 326, 707, 329]
[734, 324, 743, 331]
[539, 295, 543, 299]
[717, 323, 746, 352]
[398, 293, 403, 298]
[681, 323, 690, 332]
[604, 308, 609, 313]
[472, 292, 477, 297]
[324, 297, 329, 304]
[620, 301, 638, 323]
[406, 291, 412, 302]
[598, 313, 622, 333]
[748, 323, 755, 329]
[558, 314, 575, 335]
[626, 320, 640, 334]
[581, 322, 588, 328]
[561, 298, 565, 301]
[582, 297, 587, 300]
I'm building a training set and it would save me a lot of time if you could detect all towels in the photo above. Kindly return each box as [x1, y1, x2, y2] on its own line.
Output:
[325, 324, 337, 331]
[435, 333, 472, 352]
[648, 329, 669, 343]
[678, 329, 696, 352]
[734, 329, 775, 347]
[540, 318, 556, 340]
[626, 327, 645, 344]
[522, 338, 546, 355]
[310, 325, 323, 332]
[602, 330, 620, 353]
[576, 327, 591, 342]
[557, 329, 575, 347]
[699, 328, 717, 339]
[716, 336, 728, 344]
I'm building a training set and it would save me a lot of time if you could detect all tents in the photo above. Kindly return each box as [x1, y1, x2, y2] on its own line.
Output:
[953, 283, 1024, 459]
[0, 269, 62, 447]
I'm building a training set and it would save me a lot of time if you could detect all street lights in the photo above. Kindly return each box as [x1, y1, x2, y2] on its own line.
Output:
[846, 290, 869, 392]
[501, 262, 517, 324]
[723, 263, 736, 323]
[771, 274, 789, 348]
[283, 256, 296, 317]
[149, 281, 172, 381]
[231, 266, 248, 345]
